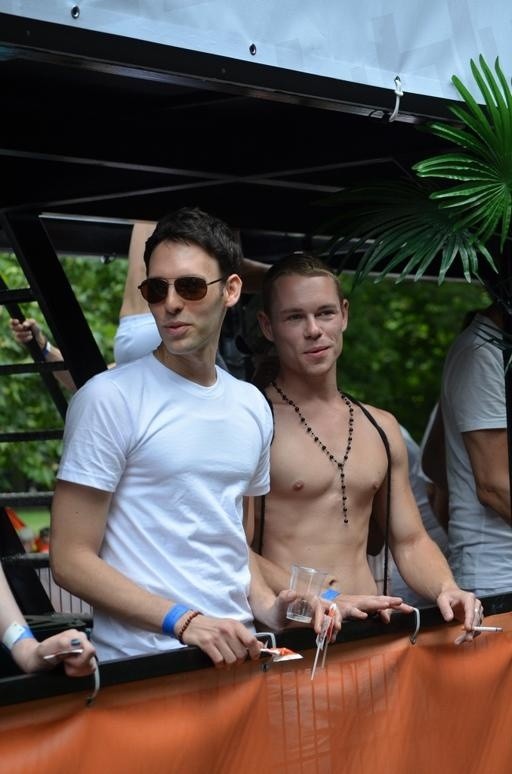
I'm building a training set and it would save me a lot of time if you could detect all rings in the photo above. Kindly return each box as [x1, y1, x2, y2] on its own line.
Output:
[474, 608, 481, 616]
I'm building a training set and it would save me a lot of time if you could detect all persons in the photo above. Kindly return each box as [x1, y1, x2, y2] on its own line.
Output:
[436, 234, 512, 598]
[111, 221, 273, 367]
[0, 558, 99, 680]
[250, 250, 490, 647]
[9, 314, 116, 396]
[361, 418, 461, 610]
[45, 203, 277, 674]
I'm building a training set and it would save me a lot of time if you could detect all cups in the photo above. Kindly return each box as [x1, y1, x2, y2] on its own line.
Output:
[285, 563, 328, 626]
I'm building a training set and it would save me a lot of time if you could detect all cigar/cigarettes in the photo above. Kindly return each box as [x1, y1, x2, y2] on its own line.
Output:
[460, 625, 504, 633]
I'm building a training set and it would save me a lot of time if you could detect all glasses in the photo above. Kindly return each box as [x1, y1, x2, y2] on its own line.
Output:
[137, 275, 223, 305]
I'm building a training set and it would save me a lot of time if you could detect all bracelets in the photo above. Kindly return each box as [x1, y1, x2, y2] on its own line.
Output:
[162, 602, 191, 640]
[1, 623, 37, 650]
[177, 610, 204, 645]
[42, 340, 52, 359]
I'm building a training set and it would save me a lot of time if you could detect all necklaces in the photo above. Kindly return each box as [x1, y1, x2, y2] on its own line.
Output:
[266, 377, 355, 524]
